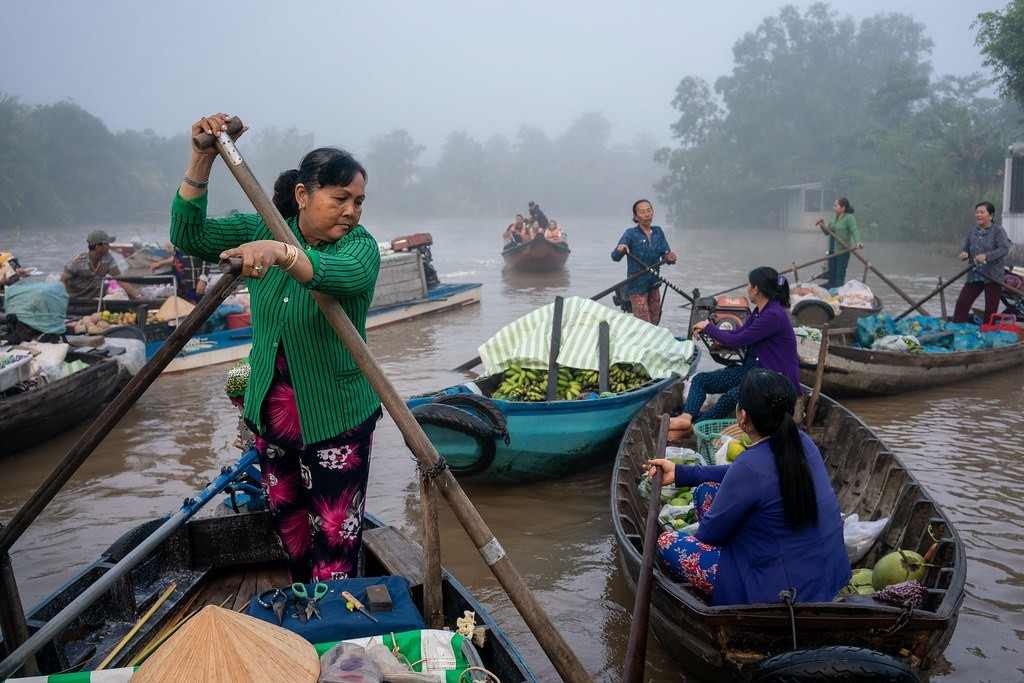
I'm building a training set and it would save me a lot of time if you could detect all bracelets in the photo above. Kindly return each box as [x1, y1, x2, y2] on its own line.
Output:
[184, 175, 209, 188]
[273, 243, 298, 271]
[136, 295, 141, 299]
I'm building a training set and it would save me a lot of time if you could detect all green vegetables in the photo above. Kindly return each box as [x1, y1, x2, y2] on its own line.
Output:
[808, 330, 819, 342]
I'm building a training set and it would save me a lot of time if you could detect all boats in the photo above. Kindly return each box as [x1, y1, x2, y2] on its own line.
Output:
[402, 294, 704, 480]
[1, 229, 484, 460]
[499, 231, 570, 274]
[609, 321, 969, 683]
[691, 286, 1024, 394]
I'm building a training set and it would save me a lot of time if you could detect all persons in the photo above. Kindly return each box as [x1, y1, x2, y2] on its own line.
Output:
[170, 112, 383, 584]
[60, 229, 144, 300]
[611, 199, 677, 326]
[641, 369, 852, 607]
[953, 202, 1009, 327]
[502, 201, 566, 251]
[151, 246, 212, 306]
[816, 197, 864, 289]
[657, 267, 803, 442]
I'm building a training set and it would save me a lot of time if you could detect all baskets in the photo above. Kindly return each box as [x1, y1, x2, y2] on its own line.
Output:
[693, 418, 749, 466]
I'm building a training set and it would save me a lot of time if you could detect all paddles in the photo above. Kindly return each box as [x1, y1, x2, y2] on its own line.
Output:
[815, 218, 931, 317]
[620, 246, 696, 301]
[194, 113, 595, 683]
[588, 253, 674, 303]
[894, 254, 982, 319]
[620, 413, 671, 683]
[679, 244, 861, 308]
[962, 253, 1024, 300]
[0, 258, 245, 548]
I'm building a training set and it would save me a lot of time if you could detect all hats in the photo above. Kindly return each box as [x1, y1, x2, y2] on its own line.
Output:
[154, 295, 196, 322]
[86, 229, 116, 245]
[126, 604, 320, 683]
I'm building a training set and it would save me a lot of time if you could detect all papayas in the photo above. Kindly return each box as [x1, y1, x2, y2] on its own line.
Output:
[727, 433, 751, 461]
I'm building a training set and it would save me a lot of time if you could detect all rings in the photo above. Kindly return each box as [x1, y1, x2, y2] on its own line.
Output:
[254, 266, 263, 274]
[201, 117, 205, 119]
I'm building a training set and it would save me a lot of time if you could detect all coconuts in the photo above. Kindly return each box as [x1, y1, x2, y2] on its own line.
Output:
[844, 550, 927, 596]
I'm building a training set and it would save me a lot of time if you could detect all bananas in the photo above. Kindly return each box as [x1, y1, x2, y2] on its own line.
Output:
[493, 364, 650, 401]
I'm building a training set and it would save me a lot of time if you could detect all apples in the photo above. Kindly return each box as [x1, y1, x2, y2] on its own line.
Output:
[645, 459, 700, 525]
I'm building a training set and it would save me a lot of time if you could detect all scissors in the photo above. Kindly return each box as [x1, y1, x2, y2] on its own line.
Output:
[256, 584, 297, 627]
[292, 582, 328, 621]
